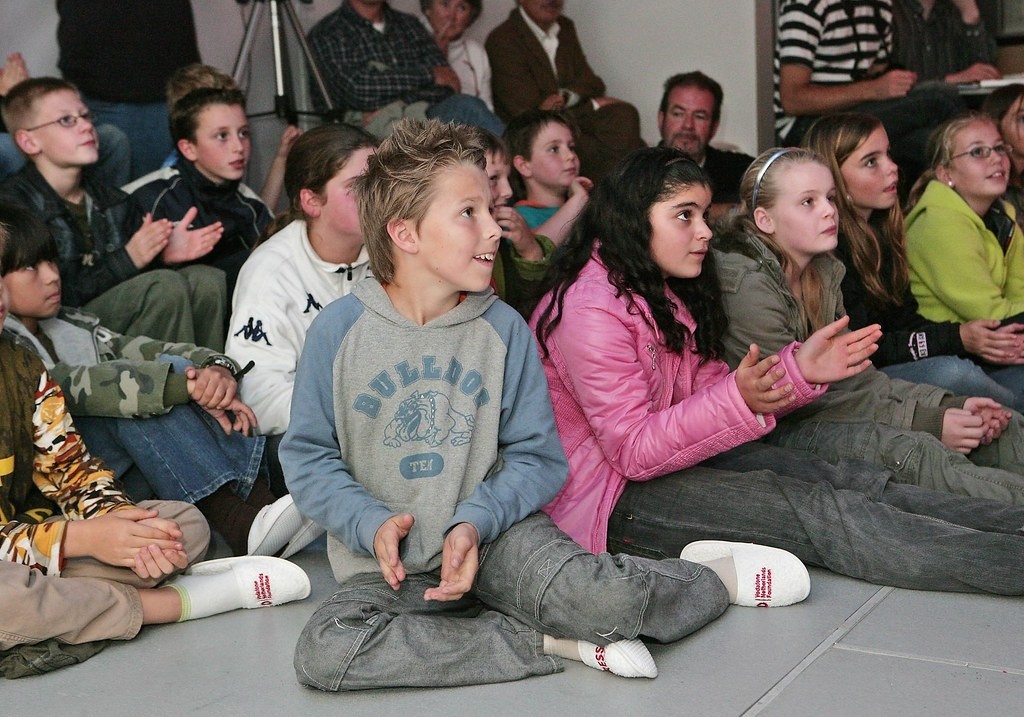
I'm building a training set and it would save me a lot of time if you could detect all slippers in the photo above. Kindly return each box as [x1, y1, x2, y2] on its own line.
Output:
[183, 556, 311, 609]
[680, 540, 811, 608]
[247, 493, 328, 560]
[578, 637, 658, 678]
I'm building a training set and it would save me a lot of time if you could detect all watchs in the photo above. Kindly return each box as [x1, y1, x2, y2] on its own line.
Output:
[203, 355, 236, 376]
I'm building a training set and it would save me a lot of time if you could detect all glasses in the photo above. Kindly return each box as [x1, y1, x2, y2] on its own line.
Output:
[26, 110, 99, 132]
[950, 142, 1012, 160]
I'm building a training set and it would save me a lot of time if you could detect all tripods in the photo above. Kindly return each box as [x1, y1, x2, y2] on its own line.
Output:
[232, 0, 337, 131]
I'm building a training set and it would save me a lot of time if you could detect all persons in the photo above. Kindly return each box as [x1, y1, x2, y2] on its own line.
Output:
[0, 277, 312, 654]
[773, 0, 961, 175]
[709, 147, 1024, 504]
[277, 117, 811, 693]
[506, 112, 593, 274]
[0, 0, 642, 353]
[801, 115, 1023, 414]
[529, 144, 1024, 598]
[1, 222, 326, 559]
[223, 124, 383, 497]
[890, 2, 1003, 83]
[900, 110, 1023, 323]
[650, 71, 758, 219]
[979, 85, 1023, 187]
[473, 127, 557, 320]
